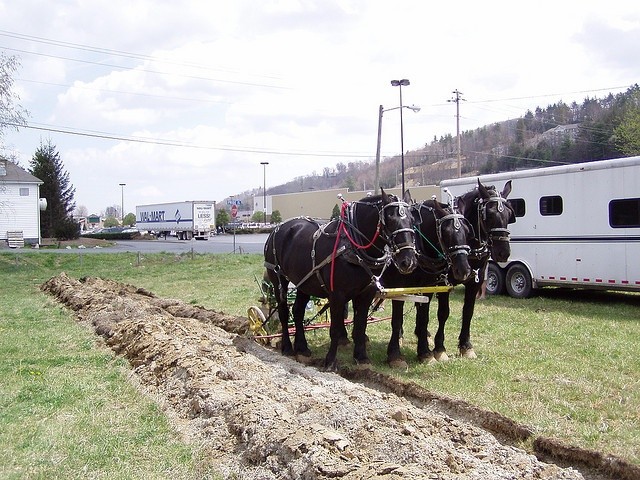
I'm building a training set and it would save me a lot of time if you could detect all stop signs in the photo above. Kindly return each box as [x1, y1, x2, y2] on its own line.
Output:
[230, 204, 237, 219]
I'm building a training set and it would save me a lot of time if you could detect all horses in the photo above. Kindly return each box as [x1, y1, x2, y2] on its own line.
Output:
[395, 178, 516, 364]
[335, 195, 472, 367]
[263, 188, 419, 375]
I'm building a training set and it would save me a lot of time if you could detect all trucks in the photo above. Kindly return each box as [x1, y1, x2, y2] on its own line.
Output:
[135, 200, 215, 240]
[440, 156, 640, 299]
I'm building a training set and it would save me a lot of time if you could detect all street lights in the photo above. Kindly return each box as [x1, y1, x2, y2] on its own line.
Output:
[390, 79, 411, 200]
[118, 183, 126, 222]
[374, 105, 421, 196]
[260, 162, 269, 223]
